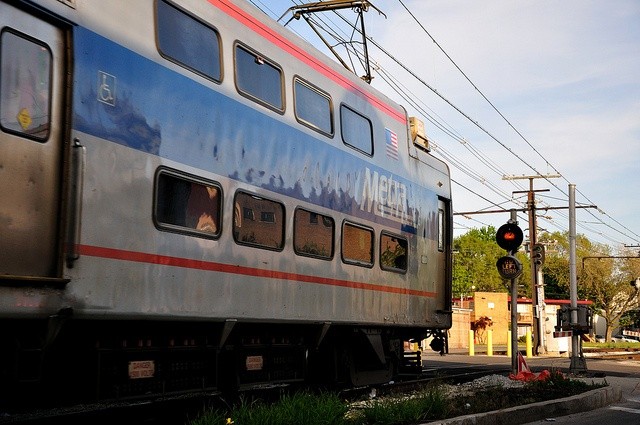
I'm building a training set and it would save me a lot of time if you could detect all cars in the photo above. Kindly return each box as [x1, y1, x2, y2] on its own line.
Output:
[627, 336, 640, 342]
[611, 335, 639, 343]
[596, 335, 605, 342]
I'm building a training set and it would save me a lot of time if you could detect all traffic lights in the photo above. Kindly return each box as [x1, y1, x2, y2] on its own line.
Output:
[496, 256, 521, 279]
[534, 243, 545, 265]
[496, 223, 523, 250]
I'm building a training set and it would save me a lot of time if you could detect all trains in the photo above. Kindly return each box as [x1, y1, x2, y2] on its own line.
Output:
[1, 0, 453, 413]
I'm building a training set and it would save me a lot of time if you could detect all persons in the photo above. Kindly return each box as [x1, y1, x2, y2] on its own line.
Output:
[439, 329, 451, 355]
